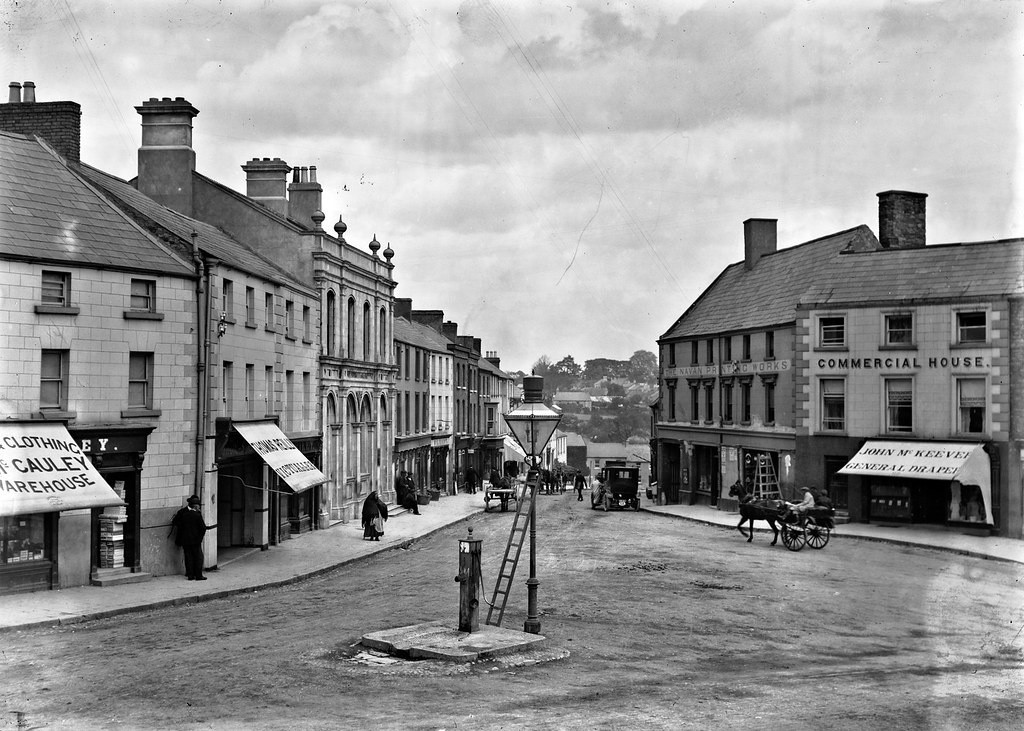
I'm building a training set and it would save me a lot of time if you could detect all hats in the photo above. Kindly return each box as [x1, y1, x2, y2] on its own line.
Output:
[410, 489, 415, 491]
[401, 471, 407, 473]
[187, 495, 204, 506]
[470, 465, 473, 467]
[577, 471, 581, 473]
[407, 472, 413, 475]
[801, 487, 809, 490]
[492, 467, 496, 470]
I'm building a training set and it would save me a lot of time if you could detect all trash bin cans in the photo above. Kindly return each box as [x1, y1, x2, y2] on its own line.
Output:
[651, 481, 657, 495]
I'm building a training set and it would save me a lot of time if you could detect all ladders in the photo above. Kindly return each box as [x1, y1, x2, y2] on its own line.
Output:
[485, 470, 544, 627]
[752, 452, 784, 501]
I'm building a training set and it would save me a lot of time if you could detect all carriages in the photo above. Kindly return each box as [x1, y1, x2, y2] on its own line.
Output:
[536, 465, 578, 494]
[483, 471, 519, 515]
[728, 479, 839, 553]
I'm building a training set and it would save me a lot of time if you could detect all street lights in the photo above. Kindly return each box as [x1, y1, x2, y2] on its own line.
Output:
[500, 366, 566, 636]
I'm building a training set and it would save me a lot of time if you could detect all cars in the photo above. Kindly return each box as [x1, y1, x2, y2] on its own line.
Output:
[589, 459, 643, 513]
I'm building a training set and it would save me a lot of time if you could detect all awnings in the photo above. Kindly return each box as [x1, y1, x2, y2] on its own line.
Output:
[0, 423, 129, 517]
[216, 421, 333, 495]
[836, 441, 985, 480]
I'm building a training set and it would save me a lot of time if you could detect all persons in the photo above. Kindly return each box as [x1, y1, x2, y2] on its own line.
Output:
[575, 471, 588, 501]
[591, 477, 604, 503]
[467, 465, 481, 494]
[489, 467, 500, 487]
[395, 471, 421, 515]
[789, 486, 831, 515]
[362, 491, 388, 541]
[172, 495, 207, 580]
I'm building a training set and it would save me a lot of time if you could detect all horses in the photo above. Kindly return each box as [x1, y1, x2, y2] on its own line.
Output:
[728, 480, 788, 546]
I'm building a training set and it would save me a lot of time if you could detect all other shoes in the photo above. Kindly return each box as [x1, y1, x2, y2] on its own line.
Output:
[375, 538, 380, 541]
[370, 538, 373, 541]
[196, 576, 207, 580]
[188, 576, 196, 580]
[413, 511, 421, 515]
